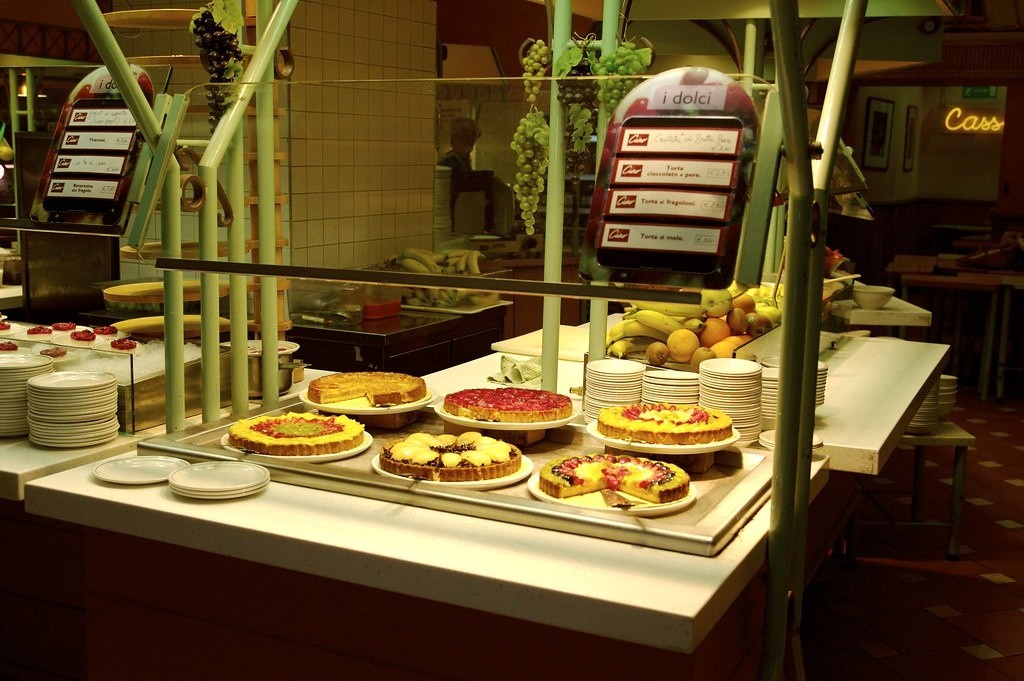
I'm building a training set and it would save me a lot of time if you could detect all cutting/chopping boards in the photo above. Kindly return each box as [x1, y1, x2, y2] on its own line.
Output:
[490, 325, 605, 362]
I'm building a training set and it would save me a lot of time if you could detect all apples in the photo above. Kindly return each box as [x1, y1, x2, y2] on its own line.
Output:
[667, 330, 700, 362]
[702, 289, 732, 316]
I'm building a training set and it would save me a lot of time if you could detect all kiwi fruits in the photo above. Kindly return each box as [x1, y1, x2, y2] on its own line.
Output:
[728, 308, 748, 334]
[761, 315, 775, 329]
[646, 343, 669, 365]
[690, 348, 716, 372]
[746, 313, 764, 336]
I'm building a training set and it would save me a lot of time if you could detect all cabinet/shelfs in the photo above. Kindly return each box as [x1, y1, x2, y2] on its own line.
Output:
[99, 9, 303, 384]
[486, 258, 583, 344]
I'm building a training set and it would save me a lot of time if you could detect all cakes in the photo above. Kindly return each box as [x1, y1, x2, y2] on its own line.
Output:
[229, 412, 365, 455]
[380, 431, 521, 481]
[444, 387, 571, 422]
[308, 371, 427, 408]
[598, 403, 732, 445]
[540, 453, 689, 504]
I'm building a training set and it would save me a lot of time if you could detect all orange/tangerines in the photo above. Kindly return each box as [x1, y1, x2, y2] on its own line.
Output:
[732, 294, 755, 313]
[701, 318, 730, 348]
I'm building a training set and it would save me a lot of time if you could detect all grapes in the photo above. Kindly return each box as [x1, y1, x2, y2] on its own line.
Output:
[508, 41, 644, 235]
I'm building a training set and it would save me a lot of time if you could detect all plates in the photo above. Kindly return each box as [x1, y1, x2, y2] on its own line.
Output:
[219, 340, 300, 357]
[642, 369, 700, 407]
[584, 359, 646, 424]
[699, 358, 762, 448]
[759, 429, 824, 452]
[905, 380, 940, 435]
[0, 351, 121, 449]
[92, 455, 190, 485]
[167, 460, 271, 499]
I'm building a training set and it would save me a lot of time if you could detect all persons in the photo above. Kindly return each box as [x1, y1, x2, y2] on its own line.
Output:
[437, 117, 481, 173]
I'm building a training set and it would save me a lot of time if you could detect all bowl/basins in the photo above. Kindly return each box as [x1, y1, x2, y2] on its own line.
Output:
[92, 341, 147, 359]
[938, 375, 958, 419]
[852, 285, 895, 309]
[0, 315, 27, 336]
[761, 360, 829, 431]
[10, 325, 131, 349]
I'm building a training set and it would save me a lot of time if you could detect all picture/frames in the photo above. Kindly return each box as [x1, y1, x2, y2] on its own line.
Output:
[903, 106, 918, 173]
[861, 96, 895, 171]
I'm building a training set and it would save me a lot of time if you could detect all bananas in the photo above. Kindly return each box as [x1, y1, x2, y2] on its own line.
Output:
[605, 301, 704, 357]
[393, 248, 499, 306]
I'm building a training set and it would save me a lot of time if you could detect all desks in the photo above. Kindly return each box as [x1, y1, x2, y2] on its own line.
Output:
[0, 299, 952, 681]
[830, 280, 932, 341]
[898, 266, 1024, 407]
[847, 415, 976, 562]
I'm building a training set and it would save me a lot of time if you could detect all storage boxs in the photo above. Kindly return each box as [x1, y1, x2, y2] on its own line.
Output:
[118, 335, 233, 433]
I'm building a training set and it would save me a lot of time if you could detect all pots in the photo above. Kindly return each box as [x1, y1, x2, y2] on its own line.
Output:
[227, 353, 312, 400]
